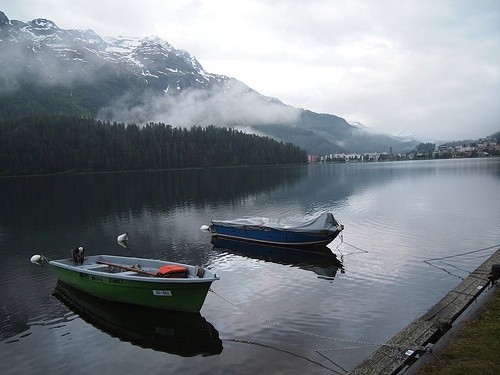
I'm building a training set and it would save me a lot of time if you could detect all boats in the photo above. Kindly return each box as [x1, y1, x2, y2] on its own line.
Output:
[200, 212, 343, 250]
[50, 280, 224, 358]
[210, 242, 339, 281]
[31, 246, 216, 312]
